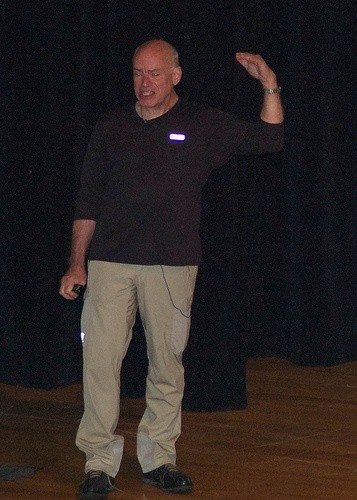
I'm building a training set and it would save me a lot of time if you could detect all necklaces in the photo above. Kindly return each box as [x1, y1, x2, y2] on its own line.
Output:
[139, 98, 171, 123]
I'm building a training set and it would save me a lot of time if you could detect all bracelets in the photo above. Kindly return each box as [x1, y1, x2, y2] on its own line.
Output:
[262, 87, 282, 93]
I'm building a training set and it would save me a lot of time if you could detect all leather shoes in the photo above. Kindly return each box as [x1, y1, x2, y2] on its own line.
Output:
[142, 462, 193, 493]
[78, 469, 116, 498]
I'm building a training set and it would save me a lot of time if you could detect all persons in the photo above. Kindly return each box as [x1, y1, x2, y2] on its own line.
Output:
[57, 41, 286, 500]
